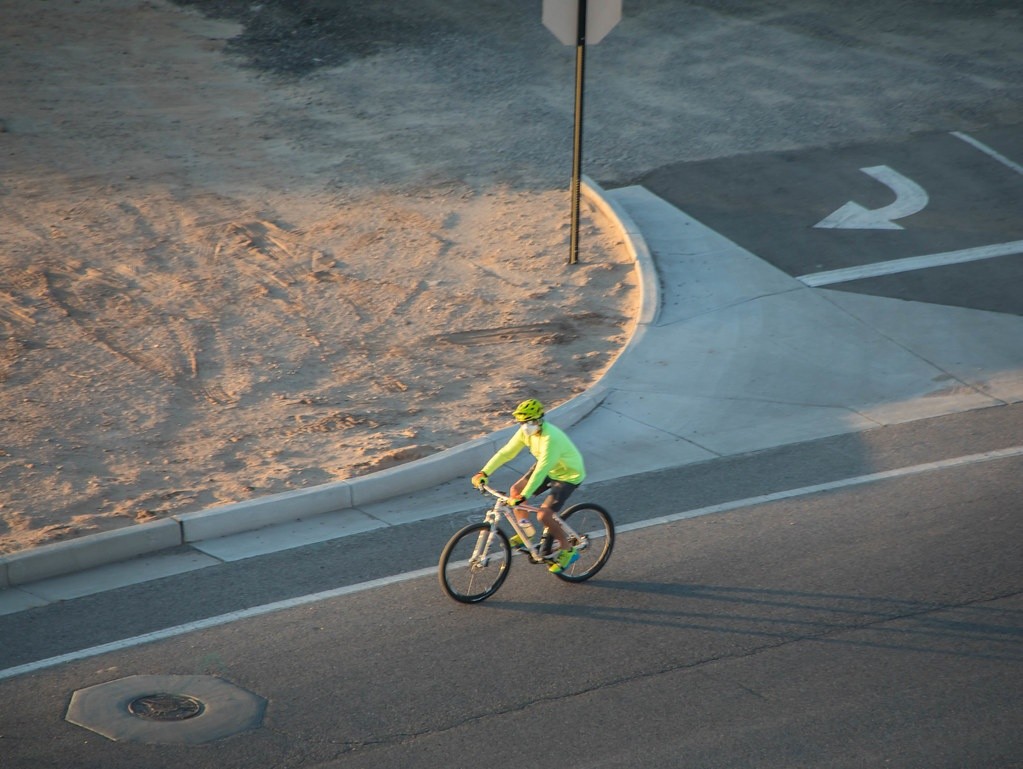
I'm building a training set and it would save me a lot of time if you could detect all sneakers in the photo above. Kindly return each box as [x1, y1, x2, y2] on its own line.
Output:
[500, 532, 535, 547]
[549, 546, 579, 574]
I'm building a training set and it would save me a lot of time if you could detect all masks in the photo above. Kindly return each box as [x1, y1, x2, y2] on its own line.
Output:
[521, 424, 540, 435]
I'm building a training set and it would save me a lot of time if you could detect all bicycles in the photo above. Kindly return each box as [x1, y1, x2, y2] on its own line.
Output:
[438, 484, 616, 604]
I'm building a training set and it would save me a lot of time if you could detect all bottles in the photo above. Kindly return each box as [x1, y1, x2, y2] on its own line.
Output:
[519, 518, 536, 537]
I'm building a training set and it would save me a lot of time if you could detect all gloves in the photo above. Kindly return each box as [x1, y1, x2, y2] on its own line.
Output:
[507, 495, 526, 505]
[472, 472, 488, 488]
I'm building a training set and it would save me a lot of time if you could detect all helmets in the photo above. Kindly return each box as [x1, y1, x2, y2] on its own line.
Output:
[512, 399, 544, 422]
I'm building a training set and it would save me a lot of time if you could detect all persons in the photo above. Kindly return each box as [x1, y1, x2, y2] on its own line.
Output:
[471, 398, 586, 573]
